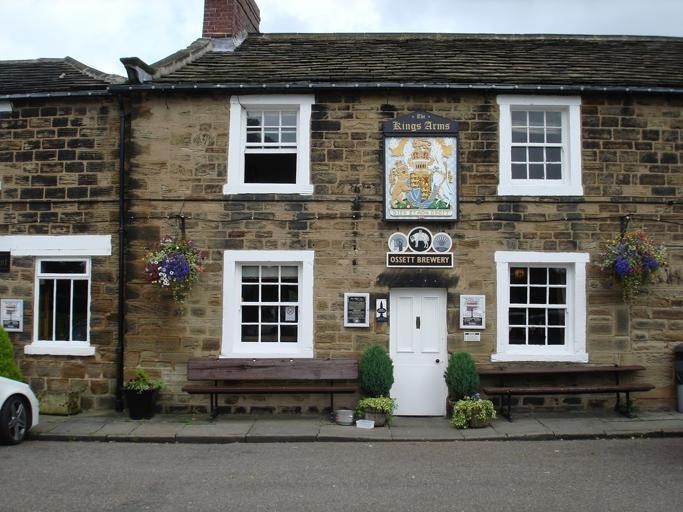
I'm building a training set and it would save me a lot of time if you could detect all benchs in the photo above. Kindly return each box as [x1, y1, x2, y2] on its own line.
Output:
[482, 383, 655, 422]
[182, 358, 360, 423]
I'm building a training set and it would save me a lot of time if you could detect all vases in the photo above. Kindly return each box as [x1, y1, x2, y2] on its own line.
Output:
[333, 410, 356, 426]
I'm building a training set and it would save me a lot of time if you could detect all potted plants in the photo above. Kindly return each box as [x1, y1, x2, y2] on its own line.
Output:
[444, 352, 480, 419]
[358, 344, 394, 424]
[355, 394, 400, 427]
[449, 393, 499, 429]
[121, 364, 165, 420]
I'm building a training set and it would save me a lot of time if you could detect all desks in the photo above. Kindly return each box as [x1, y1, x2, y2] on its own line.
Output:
[480, 364, 646, 422]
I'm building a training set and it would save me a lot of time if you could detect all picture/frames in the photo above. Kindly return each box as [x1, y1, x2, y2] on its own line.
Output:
[460, 295, 486, 330]
[344, 292, 370, 328]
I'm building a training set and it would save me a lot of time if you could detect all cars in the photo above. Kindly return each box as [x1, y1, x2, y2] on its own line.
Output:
[0, 373, 42, 445]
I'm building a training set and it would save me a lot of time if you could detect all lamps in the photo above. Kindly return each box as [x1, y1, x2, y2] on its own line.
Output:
[0, 101, 13, 113]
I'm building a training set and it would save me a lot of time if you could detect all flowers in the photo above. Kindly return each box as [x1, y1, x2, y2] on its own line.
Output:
[142, 234, 204, 317]
[591, 228, 671, 299]
